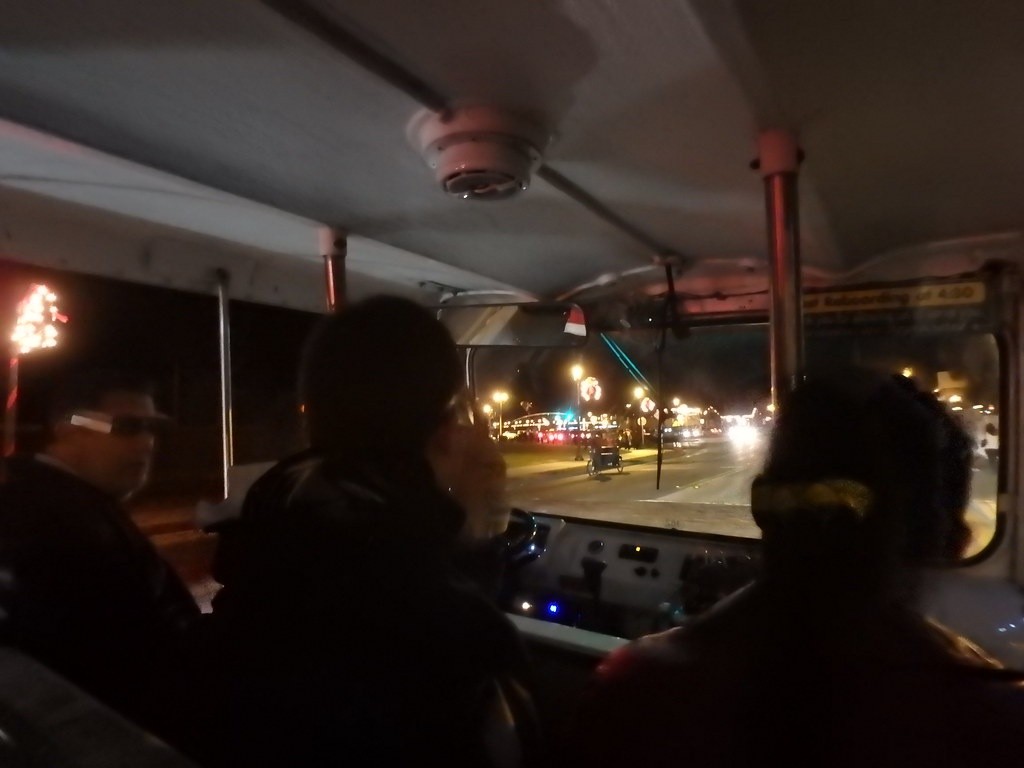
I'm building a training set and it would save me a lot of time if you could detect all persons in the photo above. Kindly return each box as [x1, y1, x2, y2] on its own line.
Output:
[204, 305, 544, 768]
[981, 423, 999, 464]
[1, 360, 203, 767]
[552, 367, 1024, 768]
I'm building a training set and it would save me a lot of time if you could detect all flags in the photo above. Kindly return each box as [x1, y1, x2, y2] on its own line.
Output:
[564, 307, 586, 336]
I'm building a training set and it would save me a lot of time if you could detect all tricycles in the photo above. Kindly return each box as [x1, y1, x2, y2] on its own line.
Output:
[586, 444, 623, 477]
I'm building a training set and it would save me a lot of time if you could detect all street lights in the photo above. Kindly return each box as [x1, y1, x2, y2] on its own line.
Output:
[492, 391, 510, 437]
[634, 387, 646, 444]
[571, 365, 585, 461]
[3, 280, 61, 460]
